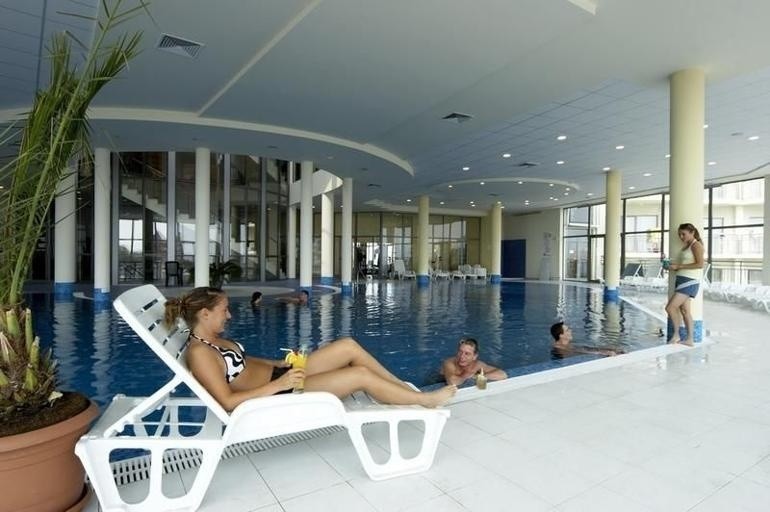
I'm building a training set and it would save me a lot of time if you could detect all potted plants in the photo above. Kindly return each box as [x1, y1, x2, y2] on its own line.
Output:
[0, 2, 139, 512]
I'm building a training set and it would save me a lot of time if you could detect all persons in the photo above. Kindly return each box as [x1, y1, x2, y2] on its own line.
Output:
[248, 290, 310, 308]
[665, 224, 704, 347]
[164, 287, 458, 411]
[430, 338, 508, 386]
[551, 322, 628, 357]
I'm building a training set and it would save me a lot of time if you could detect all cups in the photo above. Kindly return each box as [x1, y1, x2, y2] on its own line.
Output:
[284, 353, 308, 395]
[474, 372, 489, 388]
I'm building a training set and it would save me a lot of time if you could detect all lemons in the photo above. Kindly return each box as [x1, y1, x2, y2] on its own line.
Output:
[285, 352, 302, 364]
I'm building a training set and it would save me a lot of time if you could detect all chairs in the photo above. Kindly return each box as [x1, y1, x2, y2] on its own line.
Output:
[703, 281, 769, 314]
[73, 283, 454, 512]
[165, 262, 183, 286]
[617, 274, 668, 294]
[389, 260, 416, 280]
[429, 264, 487, 281]
